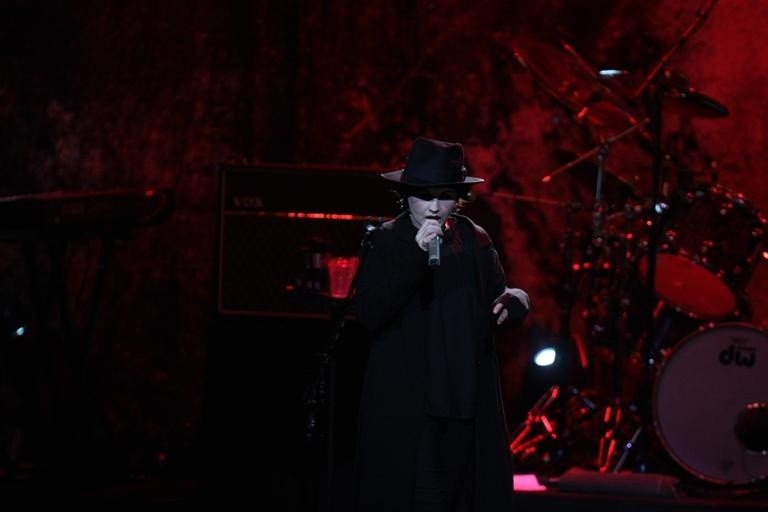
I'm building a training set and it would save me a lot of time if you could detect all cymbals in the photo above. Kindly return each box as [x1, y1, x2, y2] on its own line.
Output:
[511, 35, 729, 208]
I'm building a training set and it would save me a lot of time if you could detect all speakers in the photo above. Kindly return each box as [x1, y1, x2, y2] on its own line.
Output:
[218, 166, 391, 316]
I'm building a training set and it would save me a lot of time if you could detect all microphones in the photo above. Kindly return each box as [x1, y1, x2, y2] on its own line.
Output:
[424, 228, 444, 265]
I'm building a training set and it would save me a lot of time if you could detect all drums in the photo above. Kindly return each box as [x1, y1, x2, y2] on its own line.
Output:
[651, 321, 768, 486]
[632, 186, 766, 320]
[564, 230, 633, 320]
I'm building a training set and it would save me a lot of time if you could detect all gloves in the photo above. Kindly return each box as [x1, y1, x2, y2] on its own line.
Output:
[493, 288, 531, 321]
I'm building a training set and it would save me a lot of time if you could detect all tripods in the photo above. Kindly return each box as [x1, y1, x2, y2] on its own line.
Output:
[506, 0, 717, 476]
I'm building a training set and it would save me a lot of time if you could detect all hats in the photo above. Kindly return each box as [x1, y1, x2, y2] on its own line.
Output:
[379, 137, 486, 187]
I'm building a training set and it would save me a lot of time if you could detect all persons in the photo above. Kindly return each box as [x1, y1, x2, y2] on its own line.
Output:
[356, 136, 531, 512]
